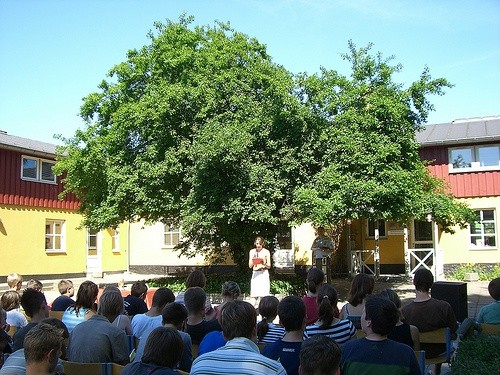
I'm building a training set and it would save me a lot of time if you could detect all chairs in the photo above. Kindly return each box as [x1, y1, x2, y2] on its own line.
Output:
[6, 310, 500, 375]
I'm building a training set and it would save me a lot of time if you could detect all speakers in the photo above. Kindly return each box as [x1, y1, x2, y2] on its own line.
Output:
[431, 281, 468, 322]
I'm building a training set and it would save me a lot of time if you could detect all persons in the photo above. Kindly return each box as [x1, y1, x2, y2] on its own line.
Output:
[248, 236, 271, 309]
[402, 269, 457, 358]
[311, 226, 336, 286]
[477, 278, 500, 325]
[0, 266, 418, 375]
